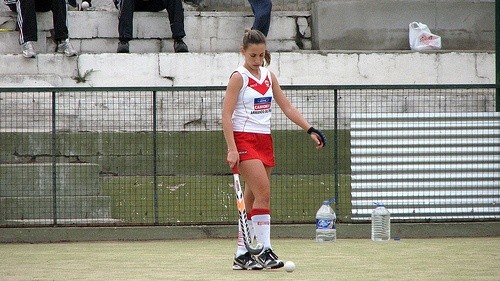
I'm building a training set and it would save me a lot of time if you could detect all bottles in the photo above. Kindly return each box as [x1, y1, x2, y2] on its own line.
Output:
[371, 202, 391, 241]
[315, 200, 336, 243]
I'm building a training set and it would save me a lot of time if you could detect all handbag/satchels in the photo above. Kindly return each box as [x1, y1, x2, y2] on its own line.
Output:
[408, 21, 440, 50]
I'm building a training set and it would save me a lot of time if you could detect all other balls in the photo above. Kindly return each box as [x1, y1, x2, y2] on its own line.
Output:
[284, 260, 295, 273]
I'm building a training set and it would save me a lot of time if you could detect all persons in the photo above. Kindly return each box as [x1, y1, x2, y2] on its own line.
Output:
[112, 0, 188, 53]
[222, 30, 326, 271]
[4, 0, 77, 59]
[248, 0, 272, 37]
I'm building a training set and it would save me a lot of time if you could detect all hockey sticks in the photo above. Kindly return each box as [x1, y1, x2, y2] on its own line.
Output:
[233, 162, 263, 255]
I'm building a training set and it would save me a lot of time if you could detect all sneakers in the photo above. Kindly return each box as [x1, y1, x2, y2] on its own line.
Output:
[58, 39, 77, 57]
[118, 41, 129, 52]
[251, 248, 284, 269]
[233, 252, 263, 269]
[174, 40, 188, 52]
[22, 41, 35, 58]
